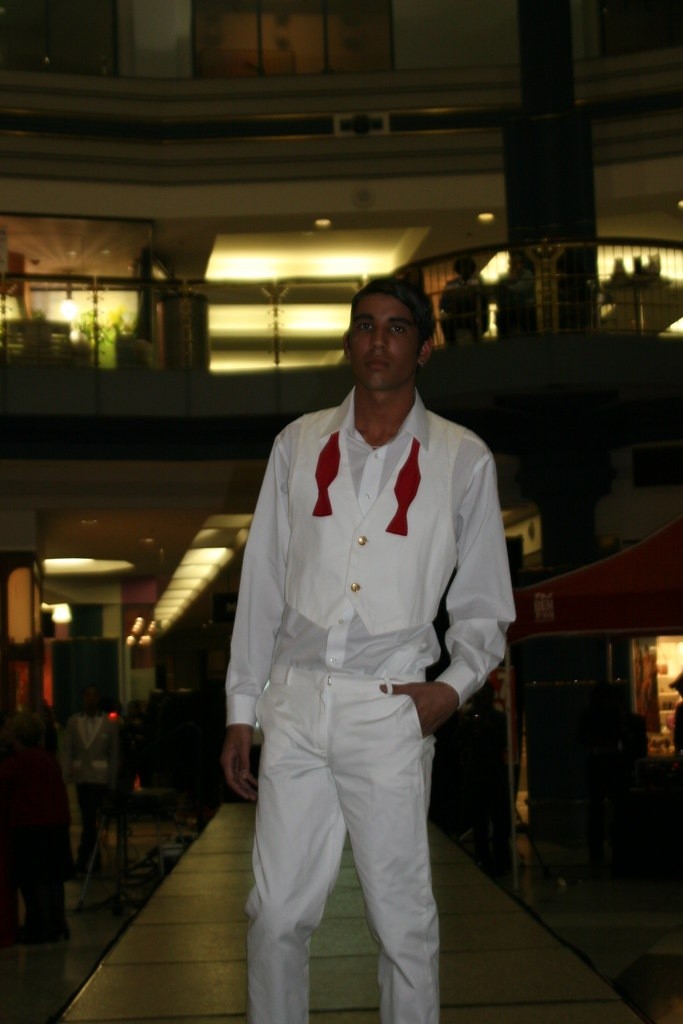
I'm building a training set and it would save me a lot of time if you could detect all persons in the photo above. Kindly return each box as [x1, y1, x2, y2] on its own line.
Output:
[0, 683, 156, 955]
[224, 277, 517, 1024]
[438, 256, 488, 343]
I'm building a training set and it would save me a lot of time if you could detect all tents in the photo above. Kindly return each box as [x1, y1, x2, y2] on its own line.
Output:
[507, 515, 683, 893]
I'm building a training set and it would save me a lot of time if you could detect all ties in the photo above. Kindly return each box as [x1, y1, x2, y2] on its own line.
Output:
[86, 718, 92, 742]
